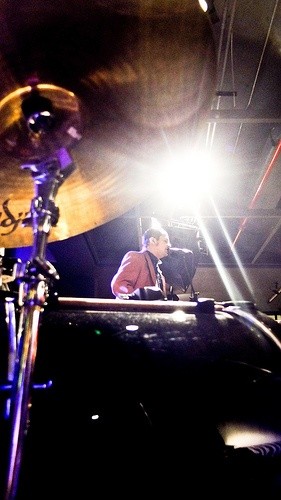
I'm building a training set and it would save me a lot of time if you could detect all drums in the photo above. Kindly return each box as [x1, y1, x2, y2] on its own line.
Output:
[0, 294, 280, 500]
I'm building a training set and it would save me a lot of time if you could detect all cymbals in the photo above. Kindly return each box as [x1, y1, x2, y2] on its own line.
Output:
[1, 1, 221, 248]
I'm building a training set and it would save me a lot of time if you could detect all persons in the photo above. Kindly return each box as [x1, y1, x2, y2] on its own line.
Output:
[110, 227, 172, 301]
